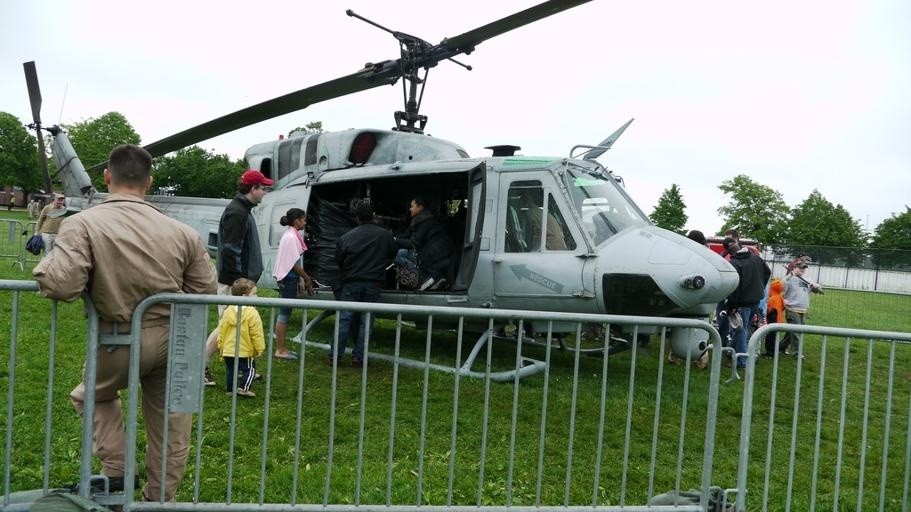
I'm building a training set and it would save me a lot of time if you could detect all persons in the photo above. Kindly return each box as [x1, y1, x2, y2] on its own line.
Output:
[204, 169, 274, 387]
[667, 230, 823, 369]
[8, 192, 44, 219]
[272, 208, 312, 359]
[328, 204, 398, 367]
[216, 277, 265, 398]
[34, 194, 69, 256]
[32, 144, 219, 503]
[391, 197, 454, 291]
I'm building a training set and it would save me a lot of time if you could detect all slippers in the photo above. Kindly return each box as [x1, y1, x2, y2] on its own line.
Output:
[275, 350, 297, 359]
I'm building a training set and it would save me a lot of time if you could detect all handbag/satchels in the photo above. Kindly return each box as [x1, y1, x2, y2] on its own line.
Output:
[396, 240, 420, 291]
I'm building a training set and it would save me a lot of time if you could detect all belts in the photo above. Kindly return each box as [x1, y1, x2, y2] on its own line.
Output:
[98, 316, 170, 333]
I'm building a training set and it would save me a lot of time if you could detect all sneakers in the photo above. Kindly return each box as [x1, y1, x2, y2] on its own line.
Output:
[204, 365, 216, 386]
[418, 274, 446, 291]
[237, 387, 255, 397]
[792, 354, 806, 360]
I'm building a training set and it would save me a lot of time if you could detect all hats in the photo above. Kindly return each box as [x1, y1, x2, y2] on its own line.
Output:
[795, 261, 807, 268]
[54, 194, 65, 199]
[241, 170, 275, 185]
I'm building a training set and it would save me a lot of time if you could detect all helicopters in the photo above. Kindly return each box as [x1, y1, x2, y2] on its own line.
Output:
[19, 1, 747, 385]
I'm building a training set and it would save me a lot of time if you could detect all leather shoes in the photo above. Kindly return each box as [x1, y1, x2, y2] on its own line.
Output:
[90, 476, 138, 492]
[328, 357, 363, 367]
